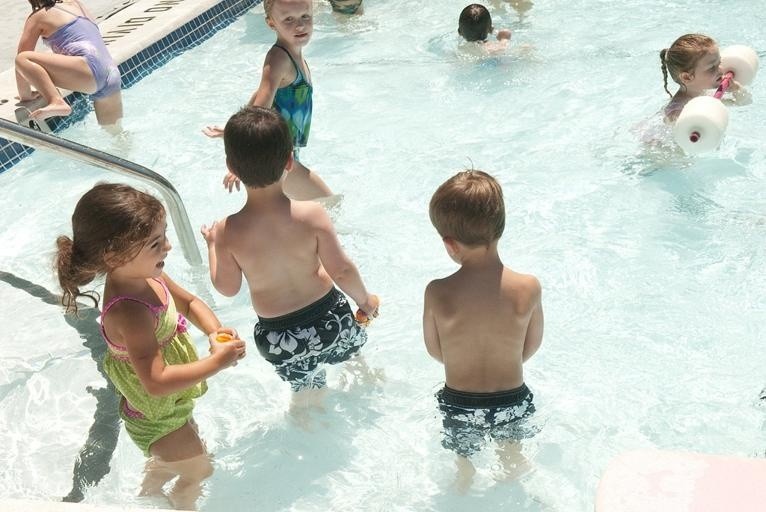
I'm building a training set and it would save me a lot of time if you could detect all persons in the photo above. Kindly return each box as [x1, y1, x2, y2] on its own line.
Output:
[420, 156, 544, 485]
[12, 0, 123, 136]
[201, 104, 380, 411]
[659, 32, 752, 122]
[201, 0, 332, 202]
[456, 3, 512, 59]
[53, 183, 246, 510]
